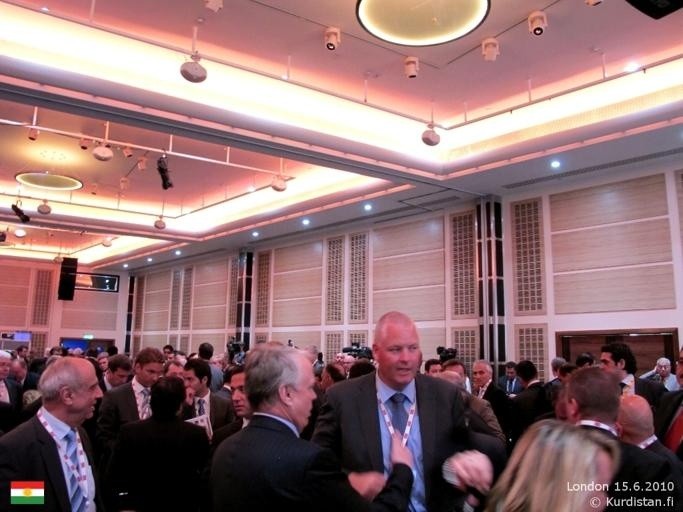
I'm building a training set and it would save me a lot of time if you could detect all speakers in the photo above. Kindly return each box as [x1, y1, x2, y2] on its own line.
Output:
[58, 257, 78, 300]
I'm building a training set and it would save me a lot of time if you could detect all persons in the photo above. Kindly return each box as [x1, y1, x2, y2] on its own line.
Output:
[612, 393, 681, 509]
[208, 337, 415, 511]
[309, 310, 509, 511]
[95, 378, 214, 512]
[0, 355, 110, 509]
[563, 365, 673, 511]
[0, 334, 682, 453]
[481, 414, 624, 512]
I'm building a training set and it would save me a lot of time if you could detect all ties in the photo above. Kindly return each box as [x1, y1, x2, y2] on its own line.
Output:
[619, 382, 628, 396]
[140, 389, 153, 420]
[0, 380, 8, 402]
[390, 392, 411, 443]
[665, 408, 683, 455]
[64, 431, 86, 512]
[197, 397, 205, 415]
[509, 379, 512, 394]
